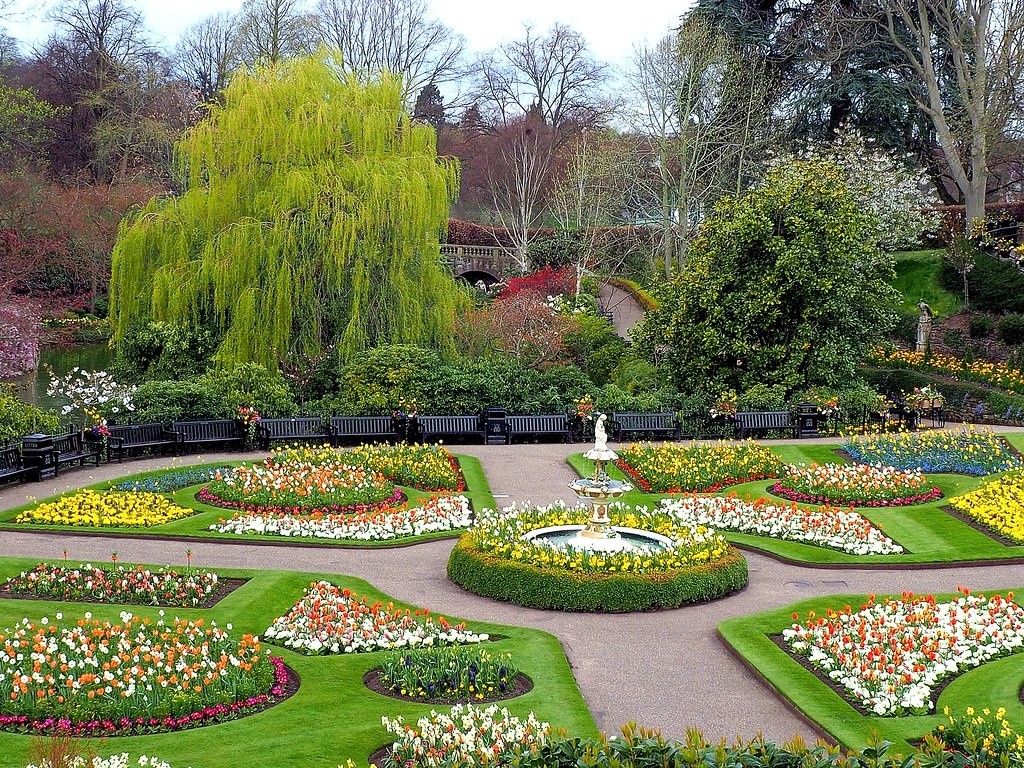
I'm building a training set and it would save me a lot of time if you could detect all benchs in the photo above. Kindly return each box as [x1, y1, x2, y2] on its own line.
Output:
[504, 413, 574, 444]
[0, 446, 42, 482]
[171, 418, 247, 451]
[610, 411, 682, 442]
[106, 420, 180, 464]
[51, 430, 104, 477]
[329, 416, 402, 447]
[257, 414, 333, 449]
[416, 413, 488, 445]
[735, 411, 802, 441]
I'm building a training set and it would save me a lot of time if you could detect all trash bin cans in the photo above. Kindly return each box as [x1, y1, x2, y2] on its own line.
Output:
[796, 403, 819, 438]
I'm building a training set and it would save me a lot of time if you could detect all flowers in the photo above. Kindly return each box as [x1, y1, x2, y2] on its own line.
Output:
[903, 383, 947, 410]
[709, 390, 738, 420]
[392, 397, 419, 421]
[571, 394, 601, 435]
[234, 407, 262, 453]
[82, 406, 111, 437]
[806, 393, 841, 416]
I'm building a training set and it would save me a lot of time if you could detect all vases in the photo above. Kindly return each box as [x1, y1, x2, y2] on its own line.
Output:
[921, 399, 941, 408]
[85, 433, 102, 441]
